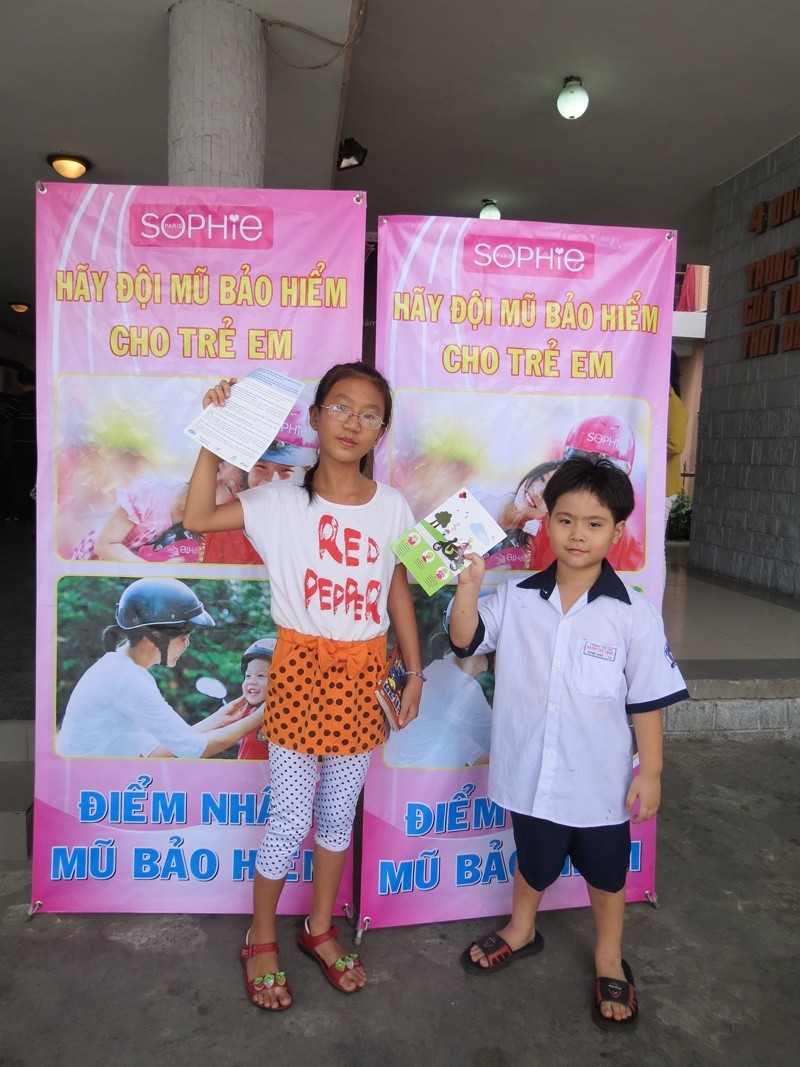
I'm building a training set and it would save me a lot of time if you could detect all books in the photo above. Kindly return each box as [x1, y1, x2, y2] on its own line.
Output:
[374, 641, 407, 732]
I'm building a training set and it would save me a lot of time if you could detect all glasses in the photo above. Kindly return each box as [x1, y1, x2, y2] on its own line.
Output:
[315, 402, 386, 430]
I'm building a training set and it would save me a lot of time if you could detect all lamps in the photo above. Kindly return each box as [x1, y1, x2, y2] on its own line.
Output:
[337, 137, 368, 172]
[46, 153, 91, 180]
[7, 301, 32, 313]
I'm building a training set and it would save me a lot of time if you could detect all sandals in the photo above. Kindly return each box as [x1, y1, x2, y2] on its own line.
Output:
[240, 927, 294, 1012]
[298, 916, 366, 994]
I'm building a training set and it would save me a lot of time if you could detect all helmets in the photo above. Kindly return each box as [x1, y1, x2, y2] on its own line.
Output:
[564, 415, 635, 475]
[260, 403, 320, 468]
[441, 586, 495, 636]
[115, 576, 216, 629]
[240, 638, 278, 675]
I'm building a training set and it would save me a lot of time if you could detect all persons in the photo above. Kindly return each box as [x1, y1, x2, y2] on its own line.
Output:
[442, 454, 692, 1035]
[55, 399, 646, 768]
[181, 361, 423, 1014]
[663, 350, 690, 599]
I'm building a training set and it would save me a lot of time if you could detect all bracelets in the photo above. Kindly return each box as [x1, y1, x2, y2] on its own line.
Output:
[406, 671, 427, 681]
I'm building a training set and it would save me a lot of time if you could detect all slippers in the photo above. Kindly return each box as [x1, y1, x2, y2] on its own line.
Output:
[460, 930, 544, 976]
[592, 958, 638, 1031]
